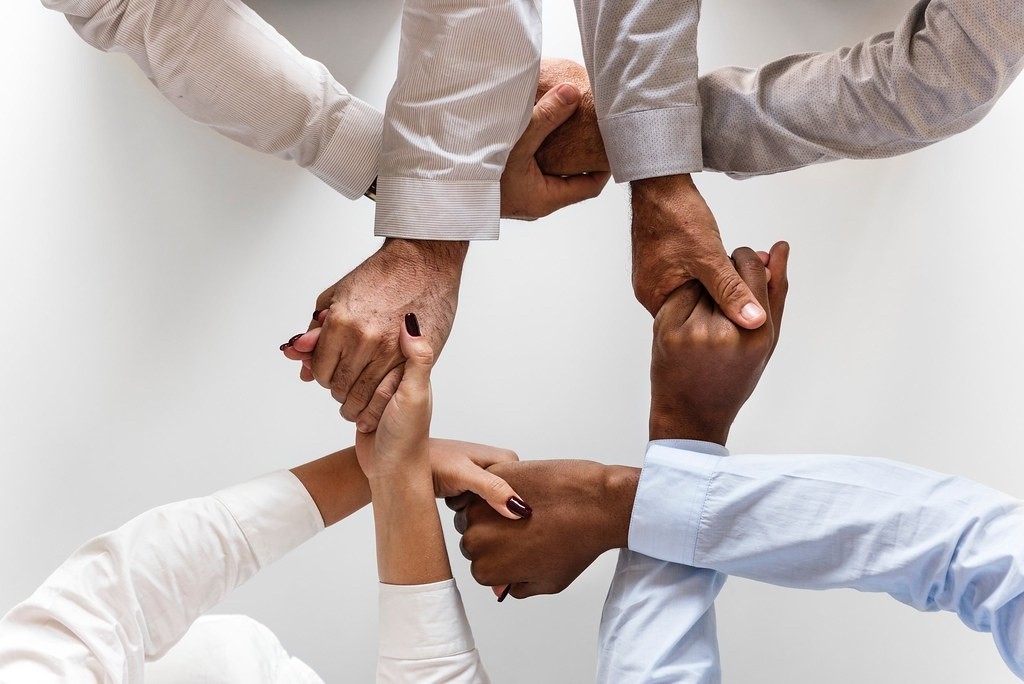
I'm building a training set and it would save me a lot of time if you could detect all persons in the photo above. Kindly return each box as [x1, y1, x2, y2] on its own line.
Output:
[1, 0, 1023, 683]
